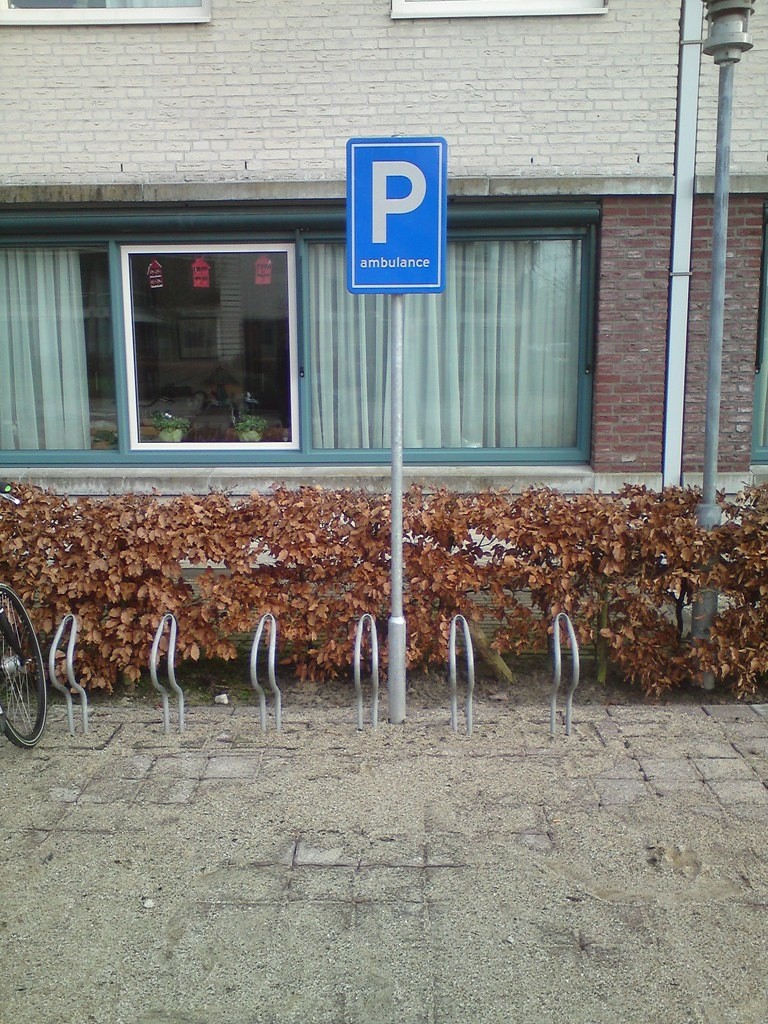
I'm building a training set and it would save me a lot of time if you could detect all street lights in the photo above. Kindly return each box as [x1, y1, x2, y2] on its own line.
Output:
[691, 0, 756, 691]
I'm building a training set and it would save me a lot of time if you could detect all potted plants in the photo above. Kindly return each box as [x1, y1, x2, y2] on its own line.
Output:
[235, 415, 267, 442]
[151, 410, 191, 442]
[93, 430, 118, 450]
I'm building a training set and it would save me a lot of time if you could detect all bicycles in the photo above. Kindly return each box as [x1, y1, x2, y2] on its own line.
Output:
[0, 481, 47, 749]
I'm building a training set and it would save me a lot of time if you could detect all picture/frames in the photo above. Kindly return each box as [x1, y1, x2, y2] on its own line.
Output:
[177, 317, 218, 359]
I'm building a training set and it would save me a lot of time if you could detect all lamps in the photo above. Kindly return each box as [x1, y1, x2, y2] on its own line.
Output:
[204, 367, 239, 406]
